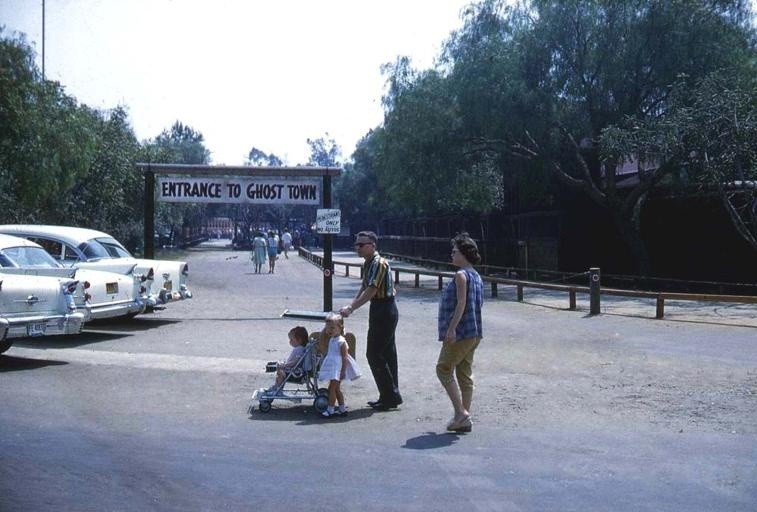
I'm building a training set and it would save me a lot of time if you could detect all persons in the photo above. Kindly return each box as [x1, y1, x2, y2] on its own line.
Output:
[251, 232, 266, 274]
[266, 230, 278, 274]
[282, 227, 292, 259]
[265, 326, 310, 396]
[339, 231, 403, 409]
[436, 239, 484, 433]
[319, 315, 362, 418]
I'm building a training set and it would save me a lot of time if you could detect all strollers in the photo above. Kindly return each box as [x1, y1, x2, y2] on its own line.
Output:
[249, 308, 346, 417]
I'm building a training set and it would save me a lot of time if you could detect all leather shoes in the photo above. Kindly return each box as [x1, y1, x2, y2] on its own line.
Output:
[373, 392, 402, 411]
[367, 399, 398, 408]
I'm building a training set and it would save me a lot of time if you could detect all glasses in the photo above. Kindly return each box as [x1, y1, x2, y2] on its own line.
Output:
[355, 243, 372, 248]
[453, 250, 460, 254]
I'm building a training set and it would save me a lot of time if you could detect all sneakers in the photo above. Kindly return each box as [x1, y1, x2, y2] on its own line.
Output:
[334, 409, 346, 415]
[447, 416, 472, 431]
[265, 386, 283, 395]
[322, 410, 334, 417]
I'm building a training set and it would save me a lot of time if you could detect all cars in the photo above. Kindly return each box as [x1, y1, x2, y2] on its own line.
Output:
[0, 232, 146, 319]
[0, 224, 193, 307]
[0, 267, 92, 354]
